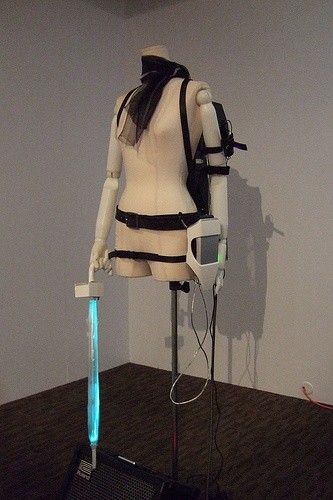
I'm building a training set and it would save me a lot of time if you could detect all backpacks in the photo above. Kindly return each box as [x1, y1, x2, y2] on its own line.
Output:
[115, 78, 247, 219]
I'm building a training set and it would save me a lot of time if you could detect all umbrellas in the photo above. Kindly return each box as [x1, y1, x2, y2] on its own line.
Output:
[86, 258, 114, 470]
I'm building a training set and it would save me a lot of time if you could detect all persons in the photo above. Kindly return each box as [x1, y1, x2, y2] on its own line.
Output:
[90, 45, 231, 297]
